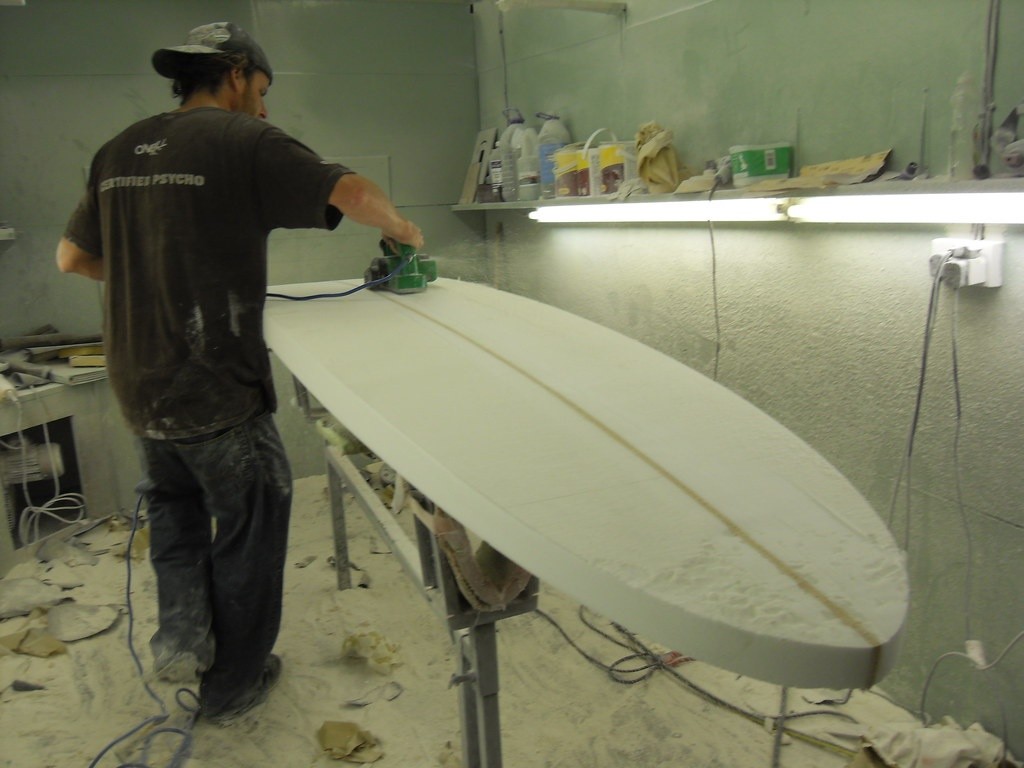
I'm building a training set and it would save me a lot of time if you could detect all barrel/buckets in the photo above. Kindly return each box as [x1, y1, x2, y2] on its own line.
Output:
[551, 125, 639, 197]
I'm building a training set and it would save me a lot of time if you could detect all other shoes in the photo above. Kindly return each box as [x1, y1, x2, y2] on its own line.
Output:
[207, 655, 281, 724]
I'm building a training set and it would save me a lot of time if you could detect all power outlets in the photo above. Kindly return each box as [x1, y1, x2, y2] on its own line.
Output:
[931, 238, 1006, 288]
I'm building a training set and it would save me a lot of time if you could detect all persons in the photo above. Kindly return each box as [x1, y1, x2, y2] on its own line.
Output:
[53, 22, 426, 728]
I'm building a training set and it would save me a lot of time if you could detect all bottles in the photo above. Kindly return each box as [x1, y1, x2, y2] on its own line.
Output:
[488, 106, 571, 201]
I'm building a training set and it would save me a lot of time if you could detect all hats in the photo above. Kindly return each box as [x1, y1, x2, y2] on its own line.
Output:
[151, 23, 272, 79]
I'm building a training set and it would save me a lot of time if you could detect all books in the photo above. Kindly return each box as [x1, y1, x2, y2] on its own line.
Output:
[0, 338, 109, 386]
